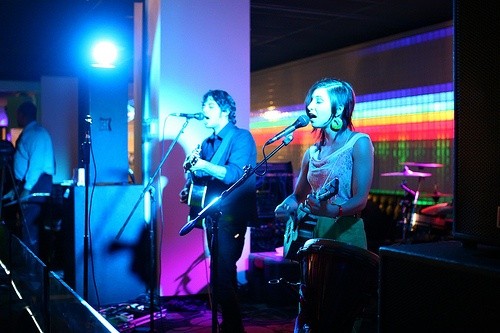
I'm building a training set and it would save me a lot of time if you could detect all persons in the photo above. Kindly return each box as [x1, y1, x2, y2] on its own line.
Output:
[273, 76, 375, 333]
[1, 102, 55, 283]
[179, 89, 260, 333]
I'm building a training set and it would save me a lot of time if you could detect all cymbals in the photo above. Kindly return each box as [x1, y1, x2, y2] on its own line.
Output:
[422, 203, 452, 216]
[399, 162, 442, 167]
[381, 168, 431, 178]
[419, 191, 455, 198]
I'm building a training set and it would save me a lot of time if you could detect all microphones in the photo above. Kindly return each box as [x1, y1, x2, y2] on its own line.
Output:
[265, 115, 310, 146]
[171, 112, 204, 120]
[401, 184, 416, 196]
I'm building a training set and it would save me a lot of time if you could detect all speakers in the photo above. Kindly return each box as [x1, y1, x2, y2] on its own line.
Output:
[377, 241, 500, 333]
[62, 184, 155, 307]
[448, 0, 500, 247]
[77, 72, 129, 185]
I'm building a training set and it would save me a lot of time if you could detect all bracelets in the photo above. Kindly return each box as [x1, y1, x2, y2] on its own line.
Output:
[333, 204, 342, 218]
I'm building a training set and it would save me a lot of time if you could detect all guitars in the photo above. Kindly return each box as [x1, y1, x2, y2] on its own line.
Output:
[282, 176, 340, 261]
[186, 142, 207, 229]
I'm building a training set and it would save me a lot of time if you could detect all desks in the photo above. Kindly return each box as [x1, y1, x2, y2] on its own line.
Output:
[377, 237, 500, 333]
